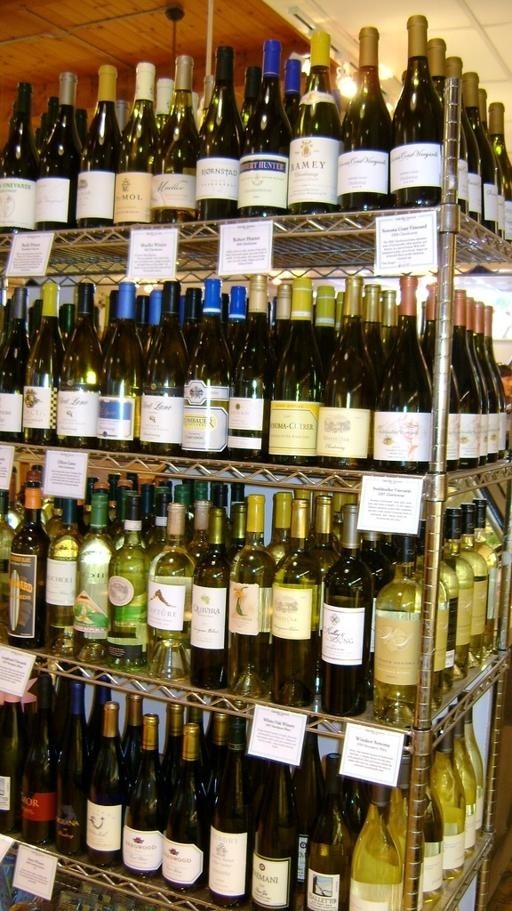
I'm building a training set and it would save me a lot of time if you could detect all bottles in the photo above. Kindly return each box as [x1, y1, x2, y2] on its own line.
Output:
[0, 467, 505, 729]
[0, 661, 489, 910]
[0, 271, 507, 471]
[0, 13, 512, 246]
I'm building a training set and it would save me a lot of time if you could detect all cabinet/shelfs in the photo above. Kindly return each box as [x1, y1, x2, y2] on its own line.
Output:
[0, 75, 512, 911]
